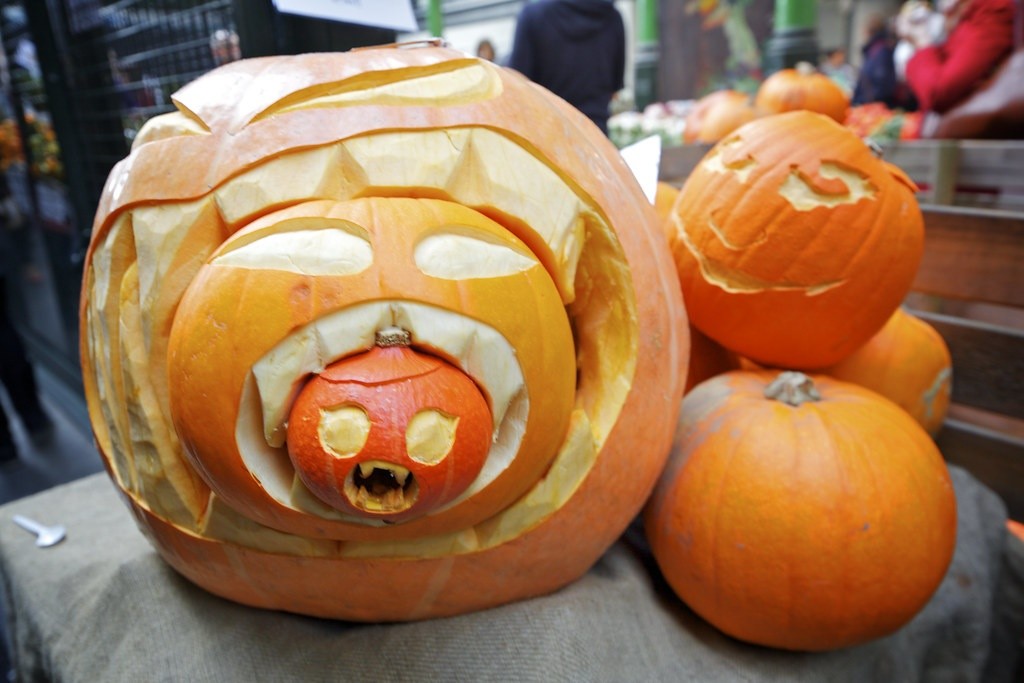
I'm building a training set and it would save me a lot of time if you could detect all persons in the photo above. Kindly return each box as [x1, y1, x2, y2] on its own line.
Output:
[850, 0, 945, 116]
[475, 39, 495, 64]
[510, 0, 627, 146]
[893, 0, 1023, 142]
[816, 9, 895, 92]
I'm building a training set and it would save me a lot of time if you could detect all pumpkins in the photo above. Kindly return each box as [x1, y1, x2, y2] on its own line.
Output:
[643, 307, 958, 653]
[755, 60, 848, 124]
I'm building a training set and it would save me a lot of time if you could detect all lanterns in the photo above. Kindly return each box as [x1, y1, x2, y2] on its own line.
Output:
[663, 110, 927, 374]
[162, 189, 579, 545]
